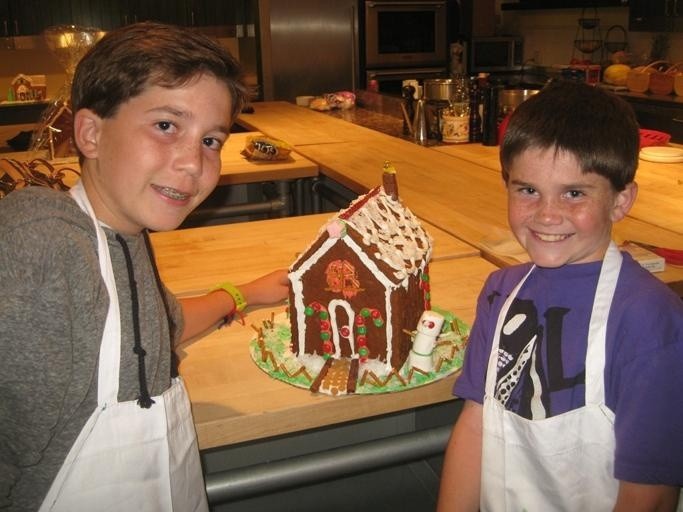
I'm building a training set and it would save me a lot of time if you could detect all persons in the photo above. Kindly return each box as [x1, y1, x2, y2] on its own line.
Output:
[436, 84, 683, 512]
[0, 23, 289, 512]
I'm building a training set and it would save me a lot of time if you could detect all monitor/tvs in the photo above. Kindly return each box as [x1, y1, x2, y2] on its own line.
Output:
[471, 37, 522, 72]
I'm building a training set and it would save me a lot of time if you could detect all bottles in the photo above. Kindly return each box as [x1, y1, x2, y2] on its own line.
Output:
[367, 74, 378, 91]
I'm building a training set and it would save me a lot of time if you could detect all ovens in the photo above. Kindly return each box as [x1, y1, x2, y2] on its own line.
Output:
[367, 2, 449, 72]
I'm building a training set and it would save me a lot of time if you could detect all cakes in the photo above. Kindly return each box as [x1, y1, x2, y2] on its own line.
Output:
[251, 159, 471, 396]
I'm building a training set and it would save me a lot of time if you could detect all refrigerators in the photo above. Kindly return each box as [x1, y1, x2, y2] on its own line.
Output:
[270, 1, 359, 97]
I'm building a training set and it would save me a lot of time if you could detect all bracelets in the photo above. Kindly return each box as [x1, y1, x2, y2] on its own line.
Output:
[206, 282, 247, 318]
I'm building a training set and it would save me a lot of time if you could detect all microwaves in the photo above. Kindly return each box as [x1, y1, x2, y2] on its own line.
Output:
[467, 38, 524, 76]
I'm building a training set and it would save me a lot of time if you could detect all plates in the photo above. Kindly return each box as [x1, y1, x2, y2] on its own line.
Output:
[640, 145, 683, 164]
